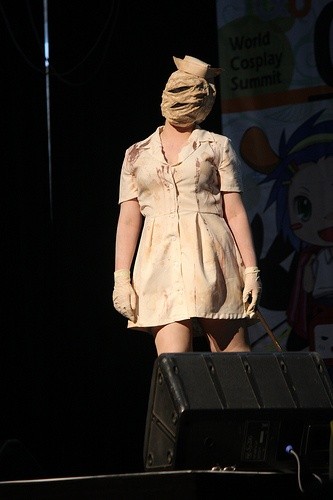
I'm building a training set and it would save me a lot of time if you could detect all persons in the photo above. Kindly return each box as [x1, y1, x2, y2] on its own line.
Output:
[114, 56, 263, 357]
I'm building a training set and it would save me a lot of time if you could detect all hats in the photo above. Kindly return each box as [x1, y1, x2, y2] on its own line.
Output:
[173, 54, 223, 81]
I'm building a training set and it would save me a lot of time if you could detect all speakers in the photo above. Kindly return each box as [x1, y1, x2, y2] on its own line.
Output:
[144, 351, 333, 471]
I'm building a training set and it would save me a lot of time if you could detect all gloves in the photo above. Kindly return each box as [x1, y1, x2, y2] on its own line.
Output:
[112, 268, 138, 323]
[241, 267, 262, 314]
